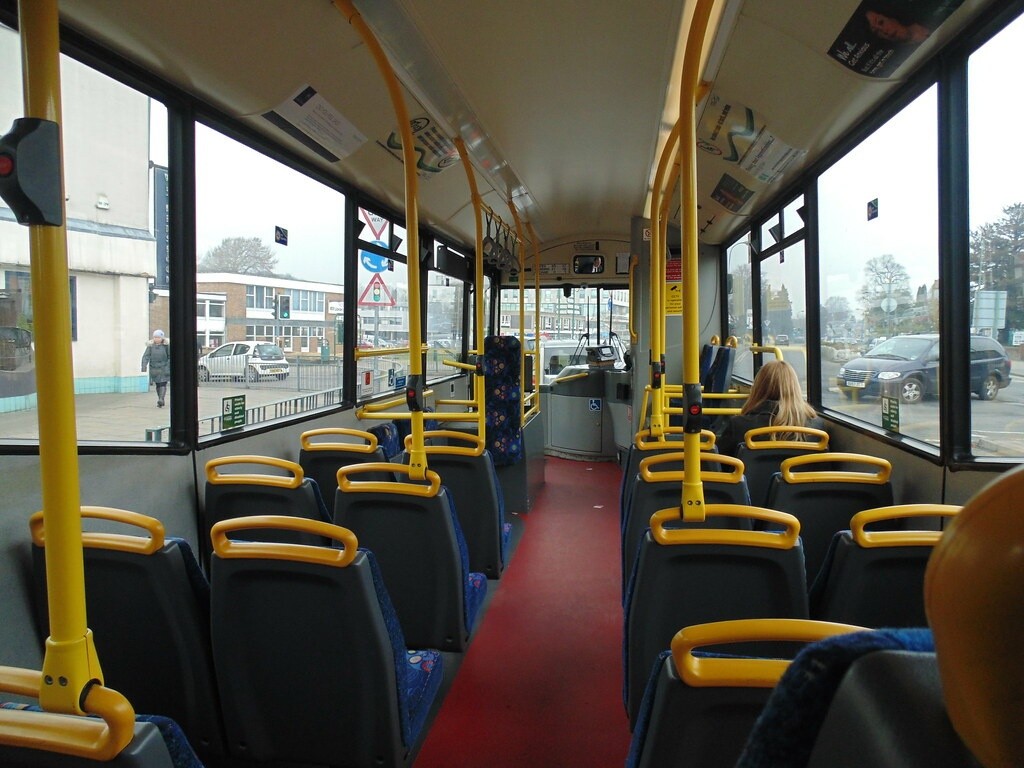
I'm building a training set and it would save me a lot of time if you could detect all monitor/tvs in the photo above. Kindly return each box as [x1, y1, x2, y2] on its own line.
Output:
[601, 348, 612, 354]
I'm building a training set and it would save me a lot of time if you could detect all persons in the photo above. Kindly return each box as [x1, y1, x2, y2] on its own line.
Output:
[716, 361, 824, 473]
[583, 257, 602, 273]
[141, 330, 170, 408]
[198, 336, 202, 360]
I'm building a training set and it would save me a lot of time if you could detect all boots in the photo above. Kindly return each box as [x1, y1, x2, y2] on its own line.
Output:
[157, 386, 165, 408]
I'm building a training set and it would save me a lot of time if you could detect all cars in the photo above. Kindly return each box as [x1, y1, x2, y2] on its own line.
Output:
[198, 340, 290, 383]
[775, 333, 790, 347]
[837, 334, 1013, 406]
[366, 337, 389, 348]
[358, 341, 374, 350]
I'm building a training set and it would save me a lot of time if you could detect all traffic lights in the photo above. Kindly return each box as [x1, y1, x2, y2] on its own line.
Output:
[271, 295, 277, 319]
[277, 295, 291, 321]
[374, 282, 382, 302]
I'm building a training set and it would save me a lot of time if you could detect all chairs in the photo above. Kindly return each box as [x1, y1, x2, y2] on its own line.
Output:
[621, 328, 1024, 768]
[0, 332, 520, 768]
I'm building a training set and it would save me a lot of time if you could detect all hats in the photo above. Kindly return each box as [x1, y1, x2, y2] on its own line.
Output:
[153, 330, 164, 339]
